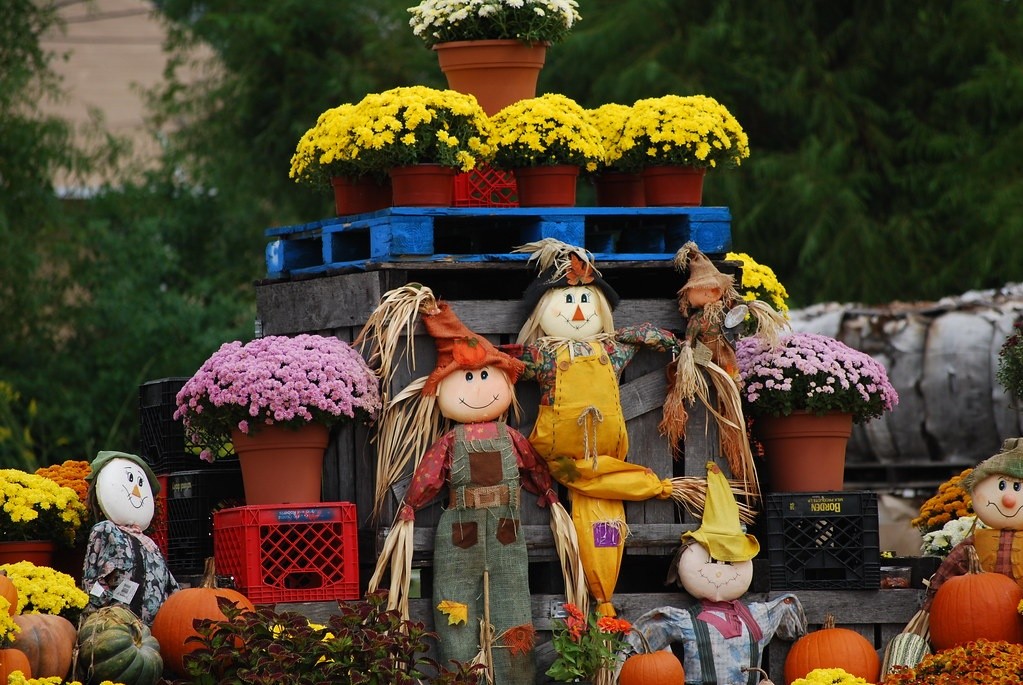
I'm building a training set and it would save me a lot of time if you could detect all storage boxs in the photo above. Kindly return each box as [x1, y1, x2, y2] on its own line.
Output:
[148, 473, 169, 560]
[168, 467, 244, 575]
[755, 487, 884, 594]
[133, 375, 238, 470]
[456, 161, 522, 207]
[211, 501, 360, 605]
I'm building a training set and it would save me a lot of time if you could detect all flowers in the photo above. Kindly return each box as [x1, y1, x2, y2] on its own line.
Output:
[0, 456, 97, 542]
[406, 0, 584, 47]
[721, 251, 791, 325]
[283, 84, 754, 181]
[169, 323, 386, 466]
[731, 325, 905, 421]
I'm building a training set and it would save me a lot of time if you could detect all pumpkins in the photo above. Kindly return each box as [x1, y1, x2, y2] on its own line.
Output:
[618, 627, 685, 685]
[783, 615, 881, 684]
[0, 555, 256, 685]
[882, 546, 1023, 685]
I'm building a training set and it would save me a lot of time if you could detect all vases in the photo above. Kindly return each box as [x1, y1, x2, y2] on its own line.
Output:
[641, 162, 712, 206]
[431, 38, 555, 117]
[592, 171, 645, 206]
[328, 174, 389, 215]
[232, 411, 332, 505]
[387, 164, 456, 209]
[516, 162, 580, 208]
[763, 403, 866, 491]
[0, 542, 53, 568]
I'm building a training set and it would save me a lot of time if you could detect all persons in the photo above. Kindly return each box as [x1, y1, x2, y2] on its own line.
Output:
[903, 438, 1023, 642]
[370, 238, 762, 685]
[610, 533, 809, 685]
[351, 281, 589, 685]
[655, 241, 794, 506]
[78, 450, 181, 628]
